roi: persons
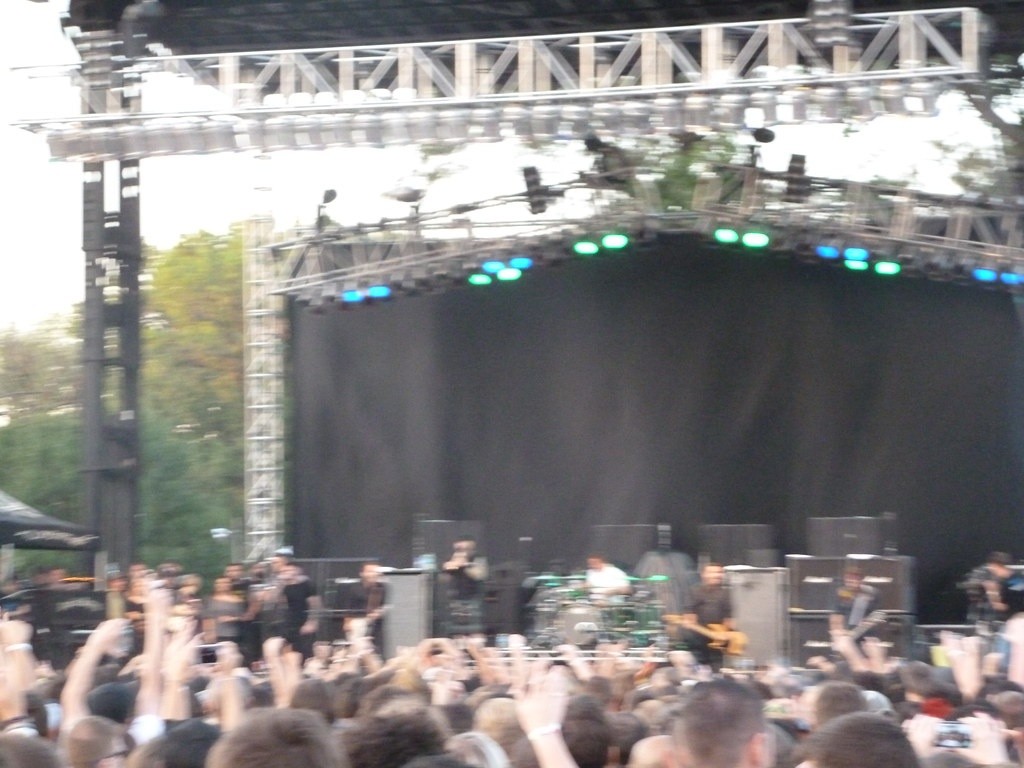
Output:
[829,566,884,645]
[0,537,1024,768]
[439,541,490,645]
[570,553,633,611]
[672,563,740,670]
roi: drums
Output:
[551,603,603,647]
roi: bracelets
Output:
[527,724,563,739]
[1,715,36,724]
[1,723,39,733]
[4,644,34,652]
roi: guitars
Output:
[831,610,889,655]
[661,614,750,655]
